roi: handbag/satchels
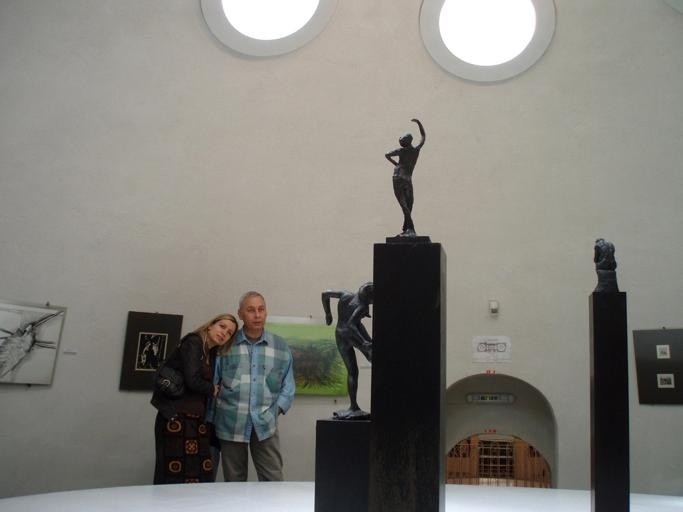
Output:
[153,364,184,399]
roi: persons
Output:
[591,236,617,274]
[205,289,296,484]
[148,313,239,487]
[320,280,371,422]
[381,115,425,240]
